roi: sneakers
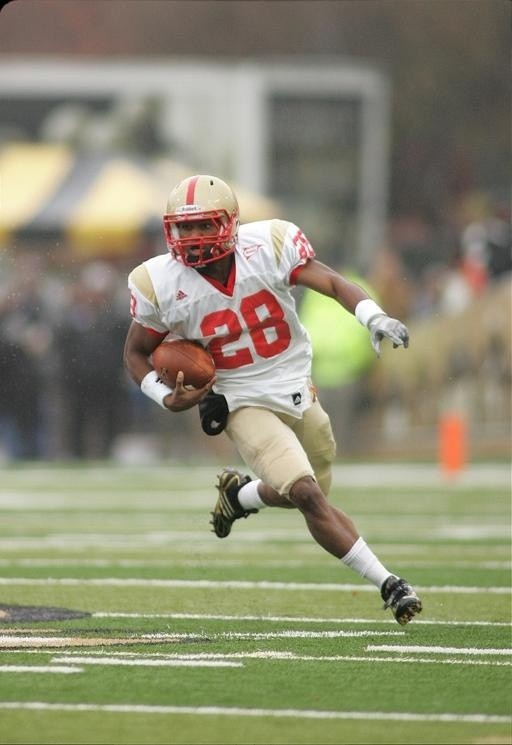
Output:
[380,576,422,627]
[209,467,259,538]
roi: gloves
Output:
[366,312,410,360]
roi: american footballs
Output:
[151,338,215,391]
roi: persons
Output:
[122,175,424,625]
[0,206,512,467]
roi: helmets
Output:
[163,175,240,268]
